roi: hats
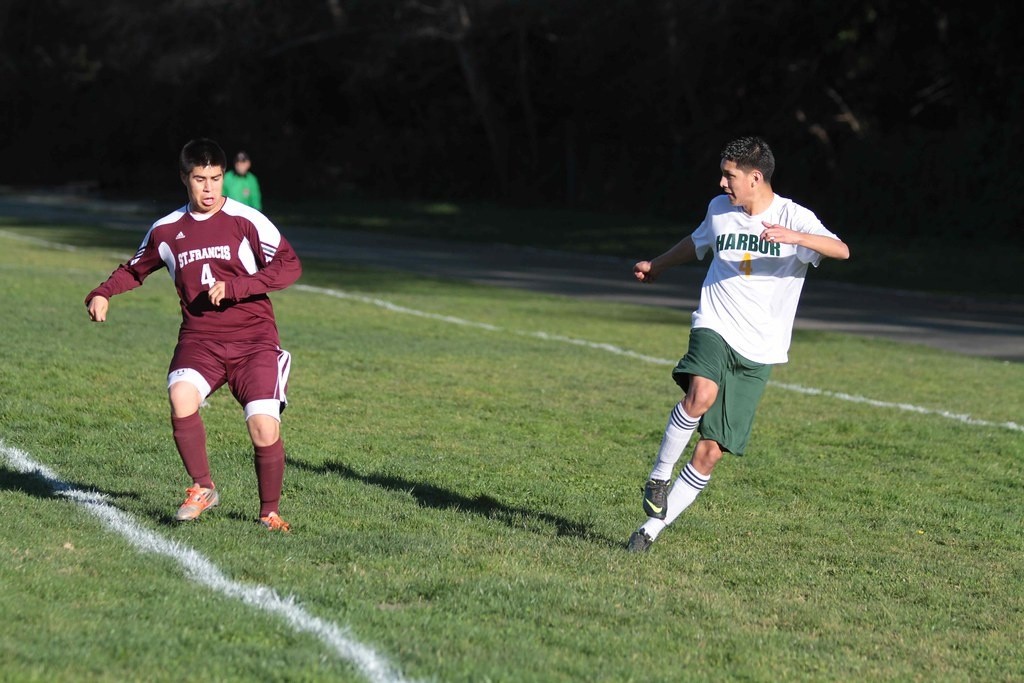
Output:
[236,152,247,160]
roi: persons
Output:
[85,139,301,532]
[221,154,263,210]
[628,137,852,547]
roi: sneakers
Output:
[174,482,219,520]
[643,478,672,519]
[259,512,289,531]
[625,528,653,552]
